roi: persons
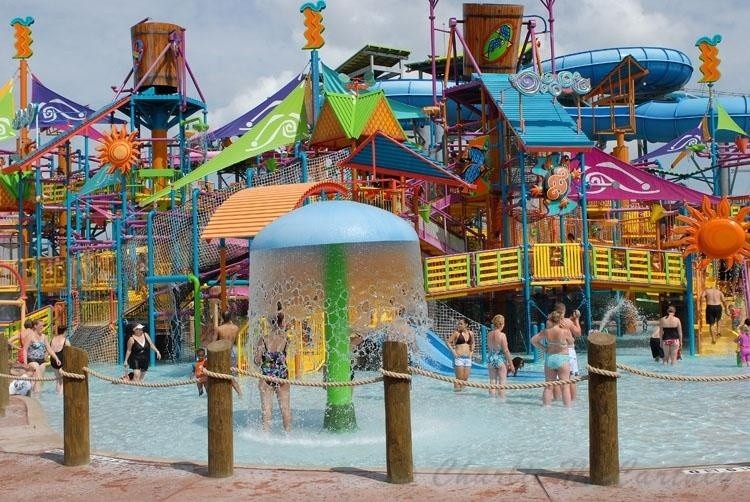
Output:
[189,349,209,396]
[8,366,37,397]
[21,319,62,392]
[649,285,750,368]
[530,310,572,405]
[506,357,524,377]
[0,157,6,170]
[447,317,475,393]
[568,233,575,243]
[8,319,35,364]
[212,309,244,397]
[253,312,291,432]
[119,365,133,382]
[546,303,582,399]
[486,314,515,399]
[50,324,71,394]
[123,323,162,382]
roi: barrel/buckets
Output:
[132,22,184,95]
[463,2,524,77]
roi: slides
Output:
[363,45,744,145]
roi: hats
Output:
[132,324,145,331]
